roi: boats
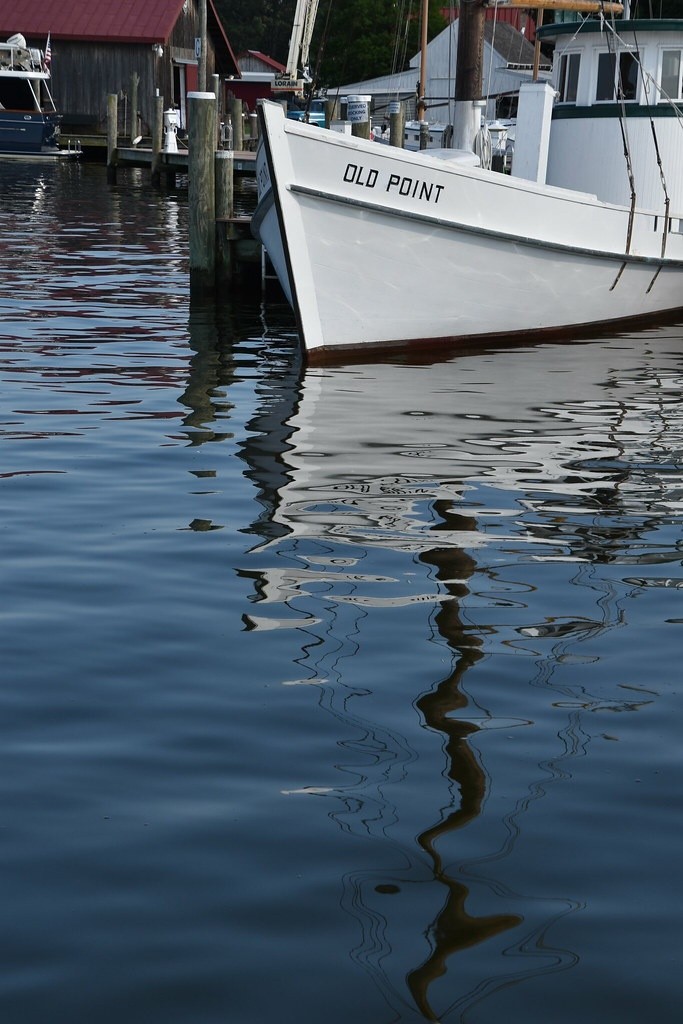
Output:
[236,300,683,634]
[250,0,683,353]
[0,30,88,161]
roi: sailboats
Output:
[370,0,531,171]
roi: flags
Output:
[45,38,51,63]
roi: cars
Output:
[288,98,330,128]
